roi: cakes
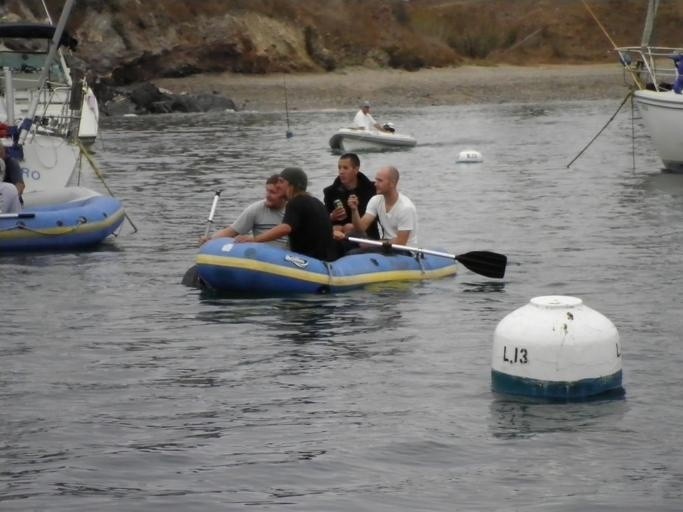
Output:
[333,198,344,209]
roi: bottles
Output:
[359,100,370,108]
[278,167,308,192]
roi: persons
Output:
[321,152,378,249]
[352,101,387,133]
[0,158,21,214]
[232,167,334,261]
[0,142,25,208]
[197,173,287,248]
[346,164,417,255]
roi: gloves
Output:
[0,186,125,250]
[0,0,99,192]
[609,0,683,173]
[196,236,457,298]
[329,127,416,152]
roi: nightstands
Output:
[342,236,507,278]
